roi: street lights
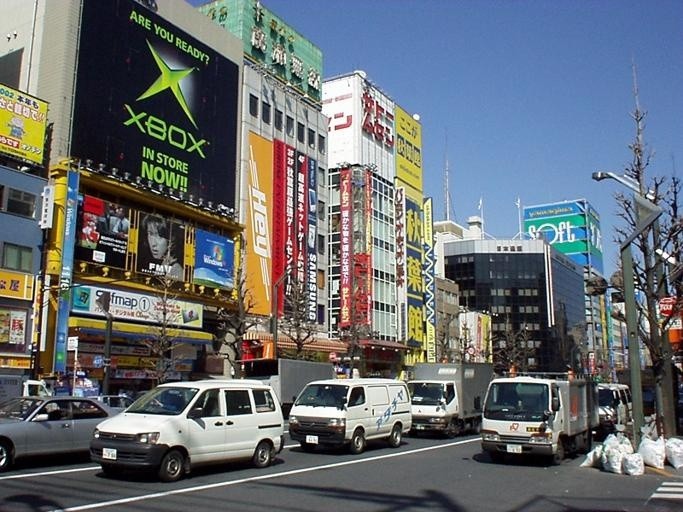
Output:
[591,168,678,448]
[446,309,468,348]
[272,263,304,358]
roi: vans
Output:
[596,382,633,427]
[88,377,287,482]
[288,378,413,456]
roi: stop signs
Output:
[329,352,336,360]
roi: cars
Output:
[82,394,135,414]
[0,395,120,473]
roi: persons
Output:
[111,207,128,234]
[140,213,183,281]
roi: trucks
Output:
[0,374,51,412]
[405,361,497,439]
[477,375,601,468]
[238,357,335,415]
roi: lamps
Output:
[71,263,224,303]
